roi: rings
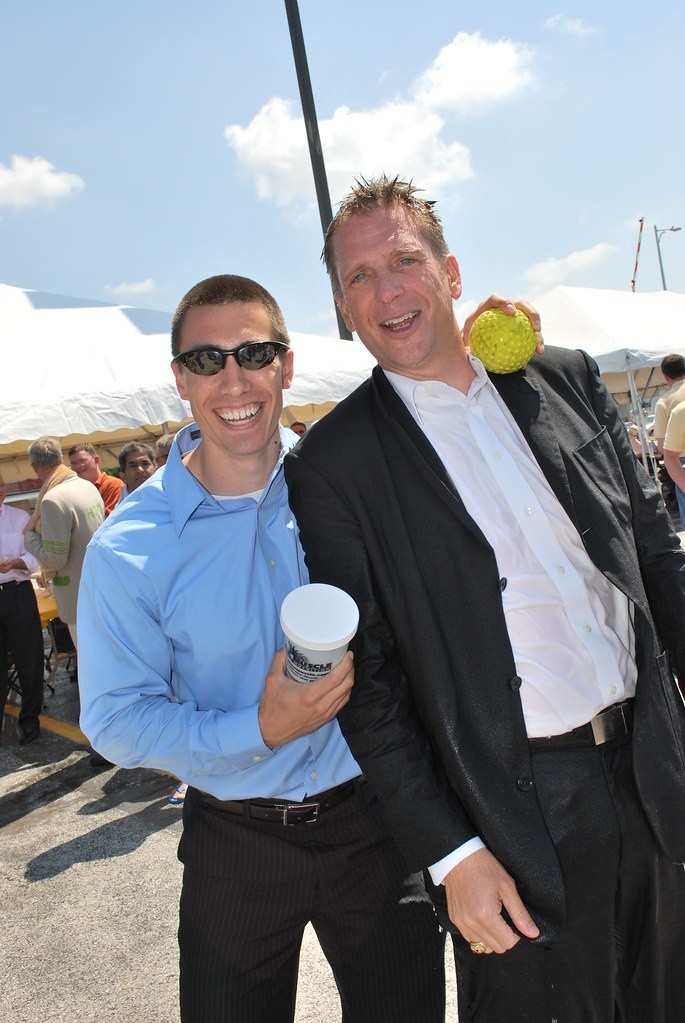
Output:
[470,941,487,954]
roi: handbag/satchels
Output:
[48,617,75,653]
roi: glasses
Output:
[151,455,168,466]
[67,457,94,470]
[173,343,290,377]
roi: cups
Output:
[280,584,362,684]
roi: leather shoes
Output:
[20,728,39,744]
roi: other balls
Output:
[467,306,539,375]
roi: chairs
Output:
[6,615,77,709]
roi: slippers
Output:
[167,785,188,803]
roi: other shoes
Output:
[91,753,109,766]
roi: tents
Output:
[530,286,685,480]
[0,281,380,484]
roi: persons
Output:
[286,175,684,1023]
[0,423,307,805]
[74,273,545,1023]
[629,354,685,529]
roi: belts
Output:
[199,776,367,828]
[0,580,29,591]
[529,699,637,748]
